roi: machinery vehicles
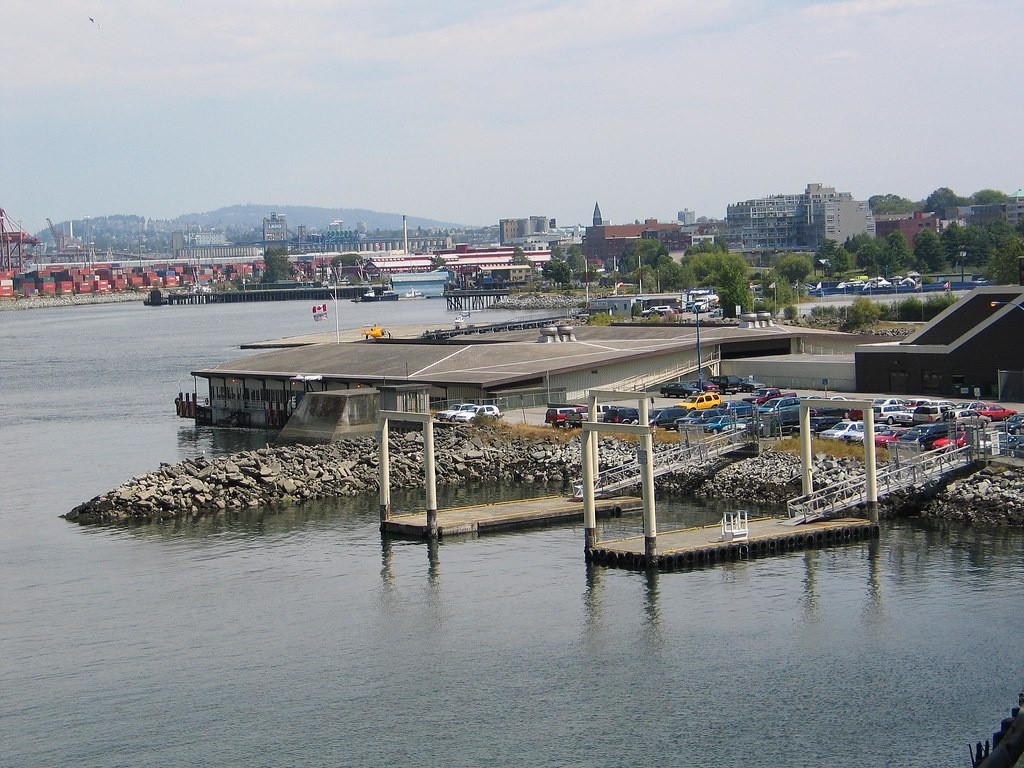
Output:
[693,295,721,312]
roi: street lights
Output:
[579,254,590,309]
[675,297,721,392]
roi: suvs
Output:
[641,305,675,317]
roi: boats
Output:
[351,284,430,303]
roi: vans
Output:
[685,300,695,313]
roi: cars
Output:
[434,403,503,424]
[544,374,1024,458]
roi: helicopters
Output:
[454,311,471,325]
[361,323,386,339]
